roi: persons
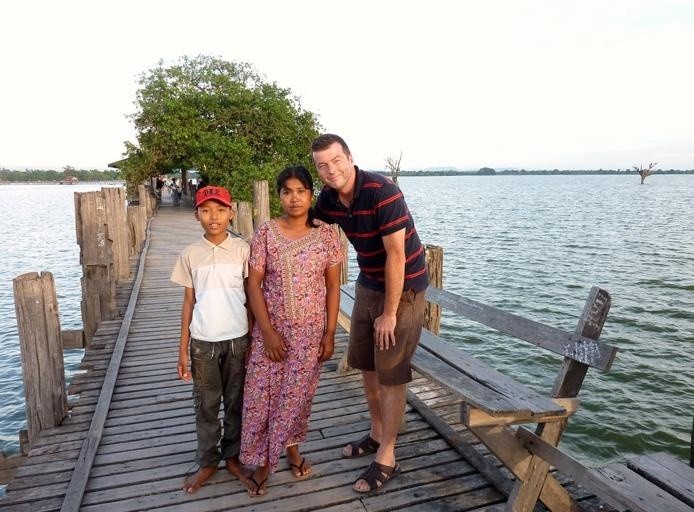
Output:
[187,180,191,187]
[155,177,165,194]
[169,176,181,206]
[305,133,433,495]
[242,165,344,499]
[168,185,255,499]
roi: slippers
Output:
[352,458,400,493]
[289,458,310,477]
[342,432,381,458]
[247,473,267,496]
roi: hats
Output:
[195,186,231,207]
